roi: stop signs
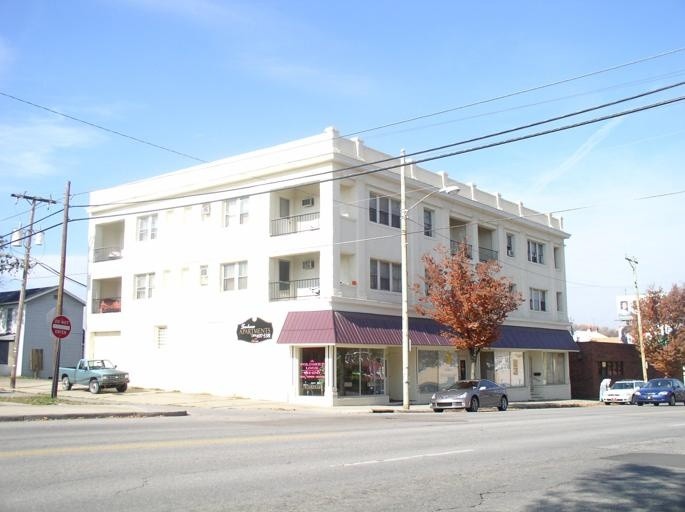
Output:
[51,315,72,338]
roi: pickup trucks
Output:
[58,358,131,395]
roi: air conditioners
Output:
[301,198,313,207]
[302,259,313,270]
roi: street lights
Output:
[399,185,462,409]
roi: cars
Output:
[419,381,445,392]
[429,378,509,413]
[602,377,685,407]
